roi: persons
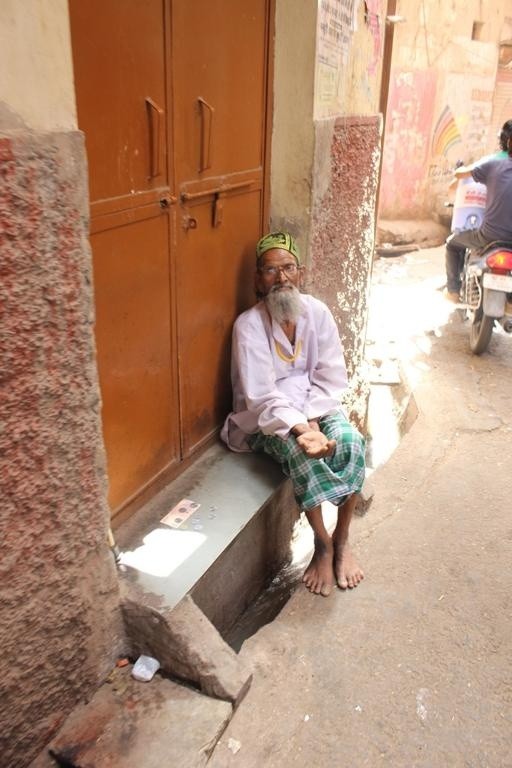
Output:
[443,124,512,319]
[218,230,368,599]
[447,118,512,190]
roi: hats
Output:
[255,231,301,268]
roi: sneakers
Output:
[504,302,512,317]
[446,293,460,303]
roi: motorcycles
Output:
[437,159,512,354]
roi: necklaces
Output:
[275,341,302,362]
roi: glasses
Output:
[260,265,298,274]
